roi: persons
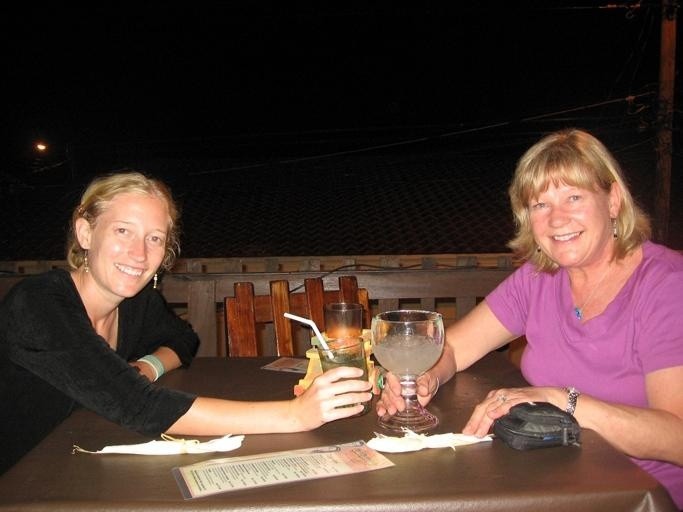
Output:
[0,173,374,471]
[375,130,683,512]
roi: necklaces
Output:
[572,258,612,320]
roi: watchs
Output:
[564,385,581,416]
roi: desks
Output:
[0,359,668,512]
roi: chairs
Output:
[224,276,368,360]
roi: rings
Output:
[496,395,506,401]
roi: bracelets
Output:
[136,354,164,383]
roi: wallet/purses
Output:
[489,400,581,450]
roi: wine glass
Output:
[369,309,445,437]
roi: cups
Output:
[323,302,362,340]
[317,336,372,419]
